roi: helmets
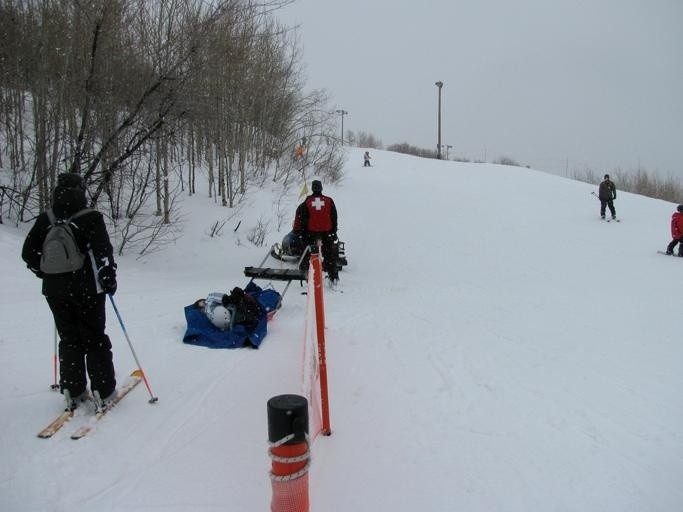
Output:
[677,204,683,211]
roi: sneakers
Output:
[67,383,86,401]
[89,380,117,400]
[328,271,339,282]
[601,215,616,219]
[666,249,683,257]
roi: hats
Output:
[311,180,323,191]
[56,172,86,191]
[604,174,609,179]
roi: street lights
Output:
[446,144,451,159]
[434,80,444,159]
[334,109,350,146]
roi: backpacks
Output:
[39,207,102,275]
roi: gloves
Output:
[27,258,43,279]
[97,258,117,296]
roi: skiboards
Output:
[36,369,144,440]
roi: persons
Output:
[21,172,117,410]
[293,180,340,285]
[666,205,683,257]
[364,152,372,166]
[194,287,264,333]
[599,175,616,219]
